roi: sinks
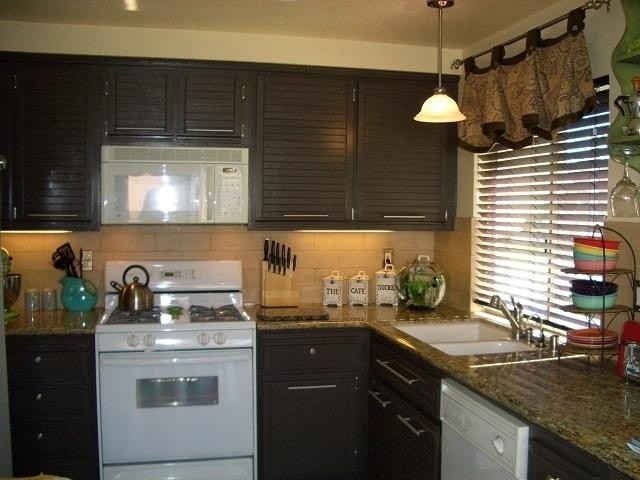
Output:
[431,342,539,356]
[393,321,512,342]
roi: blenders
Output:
[0,246,24,319]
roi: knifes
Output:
[262,237,297,274]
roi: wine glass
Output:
[608,146,640,217]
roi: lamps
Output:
[411,1,467,123]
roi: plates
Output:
[566,328,618,349]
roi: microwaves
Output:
[101,144,249,225]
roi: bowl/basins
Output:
[567,277,618,309]
[571,236,621,270]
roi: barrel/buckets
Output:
[615,320,640,382]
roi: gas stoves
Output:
[95,294,256,352]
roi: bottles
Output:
[25,287,57,313]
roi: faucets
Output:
[525,313,545,347]
[489,296,528,339]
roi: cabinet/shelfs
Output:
[526,422,639,480]
[256,329,364,480]
[1,50,102,234]
[547,216,638,376]
[101,54,252,148]
[605,0,640,174]
[363,334,440,479]
[4,331,95,480]
[251,61,460,232]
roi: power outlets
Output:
[385,254,392,265]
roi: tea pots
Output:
[109,263,155,310]
[614,74,640,135]
[58,275,98,311]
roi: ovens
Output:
[98,345,257,480]
